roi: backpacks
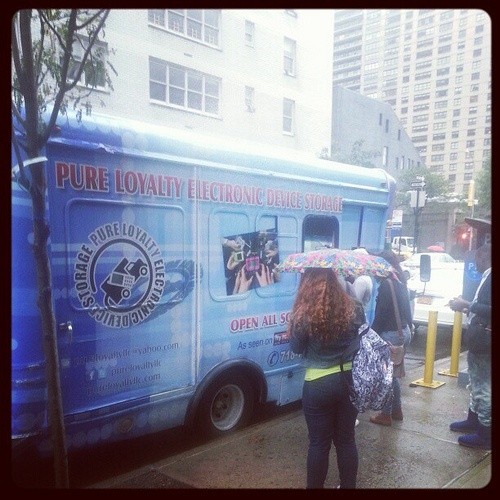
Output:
[339,299,395,412]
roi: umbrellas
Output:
[428,246,445,251]
[465,217,491,233]
[274,249,399,280]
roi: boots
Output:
[449,407,481,433]
[458,421,492,448]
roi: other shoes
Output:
[391,407,404,420]
[370,412,391,425]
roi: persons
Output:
[446,244,491,448]
[339,250,413,426]
[287,266,366,489]
[223,236,278,295]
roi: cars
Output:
[387,235,421,257]
[408,261,474,343]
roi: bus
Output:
[9,103,398,465]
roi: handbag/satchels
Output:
[389,344,406,378]
[463,323,492,357]
